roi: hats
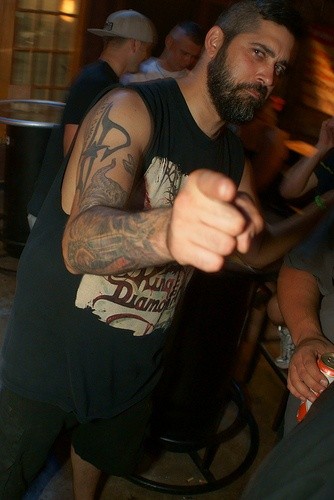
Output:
[87,9,155,42]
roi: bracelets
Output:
[315,195,325,209]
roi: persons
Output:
[0,0,309,500]
[27,9,157,231]
[239,208,334,500]
[120,22,205,85]
[227,93,291,186]
[272,116,334,221]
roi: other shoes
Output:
[275,324,295,369]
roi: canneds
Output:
[295,353,334,425]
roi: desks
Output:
[0,100,66,276]
[128,199,287,494]
